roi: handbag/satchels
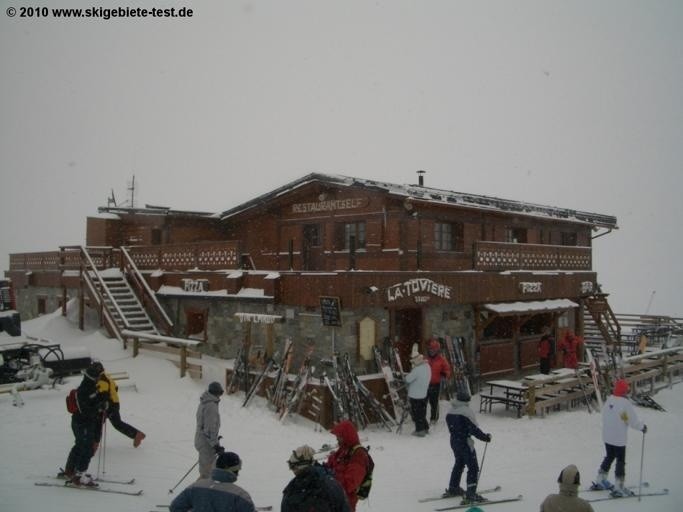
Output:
[65,389,83,417]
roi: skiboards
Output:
[155,504,273,511]
[575,329,673,415]
[226,336,473,433]
[34,478,143,495]
[419,486,523,512]
[579,482,669,503]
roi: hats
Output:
[409,352,423,365]
[288,444,316,476]
[427,340,441,353]
[208,381,224,396]
[215,451,243,473]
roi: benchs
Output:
[480,350,683,420]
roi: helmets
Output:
[612,377,627,397]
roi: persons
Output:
[445,392,491,501]
[194,382,224,477]
[638,329,649,352]
[422,339,452,423]
[557,329,584,368]
[324,420,374,512]
[540,465,596,512]
[281,445,353,512]
[169,452,257,512]
[90,361,145,455]
[537,326,557,374]
[65,365,111,485]
[595,380,649,498]
[401,351,432,437]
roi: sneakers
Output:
[63,470,97,486]
[612,486,635,498]
[409,429,430,438]
[463,492,488,502]
[445,486,465,495]
[594,477,613,490]
[133,429,145,447]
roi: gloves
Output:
[642,425,647,434]
[485,433,492,442]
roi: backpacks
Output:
[344,444,374,501]
